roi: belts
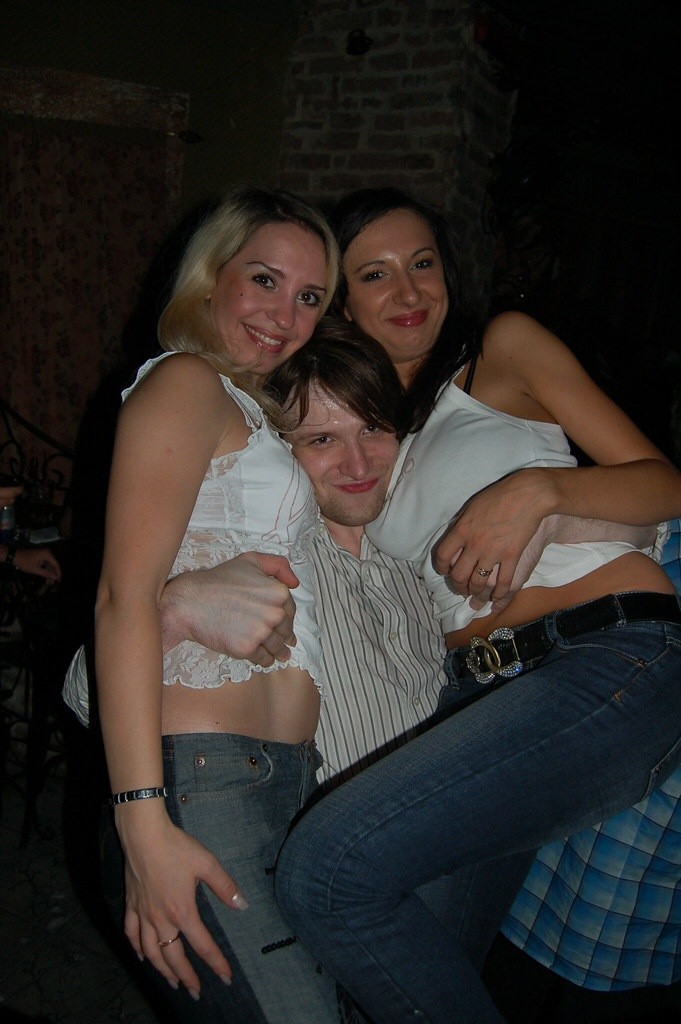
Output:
[452,593,681,684]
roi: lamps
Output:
[346,29,373,55]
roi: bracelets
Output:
[110,787,168,806]
[5,543,17,566]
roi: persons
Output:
[60,182,681,1024]
[0,486,62,581]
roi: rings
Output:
[474,566,492,577]
[158,934,180,946]
